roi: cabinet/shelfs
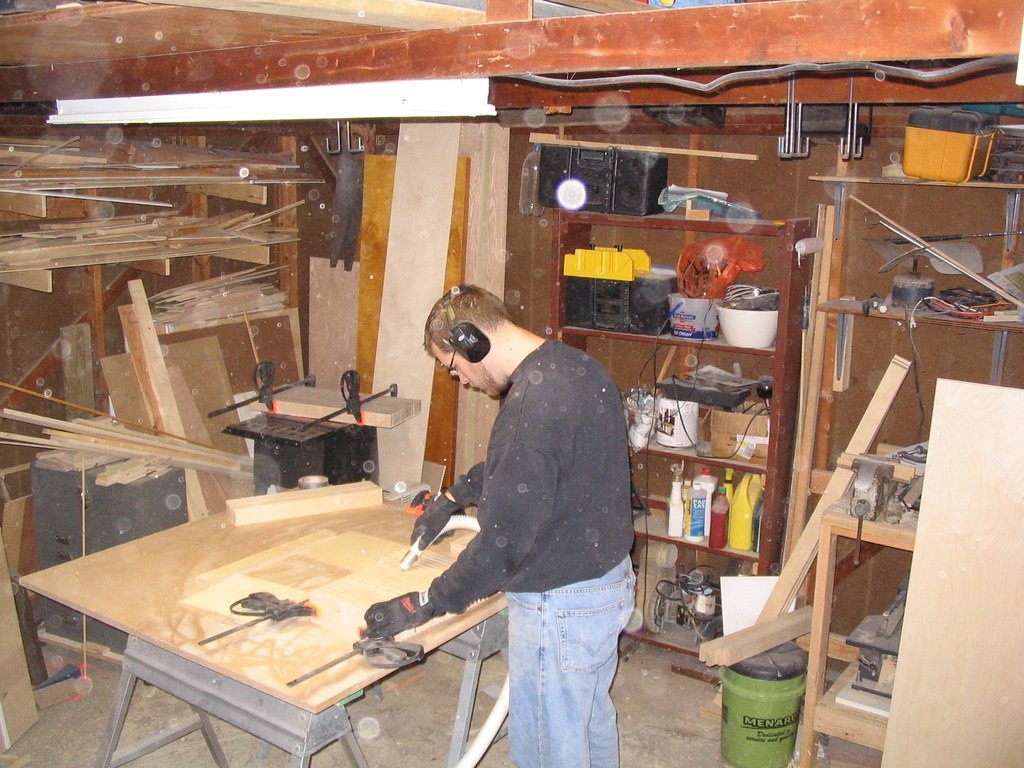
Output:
[546,209,812,657]
[794,105,1024,663]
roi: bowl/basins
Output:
[716,303,778,350]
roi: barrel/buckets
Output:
[669,292,722,341]
[719,641,809,768]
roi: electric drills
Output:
[286,628,424,692]
[196,590,322,647]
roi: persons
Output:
[362,283,637,768]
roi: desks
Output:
[18,484,509,768]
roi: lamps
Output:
[47,78,497,123]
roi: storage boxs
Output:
[902,105,1000,183]
[712,401,770,465]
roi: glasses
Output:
[448,350,460,377]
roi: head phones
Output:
[440,290,490,363]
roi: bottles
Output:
[669,462,767,552]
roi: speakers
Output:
[539,146,575,208]
[629,274,678,336]
[565,275,595,329]
[612,150,668,217]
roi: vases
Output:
[716,303,779,348]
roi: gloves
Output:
[409,493,460,551]
[363,587,435,638]
